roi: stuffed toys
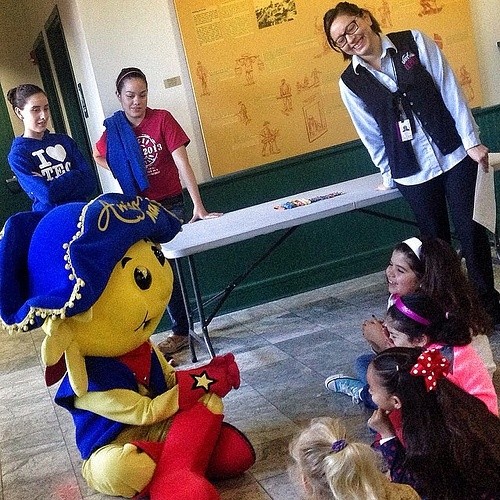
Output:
[1,195,256,500]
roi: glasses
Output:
[333,15,359,49]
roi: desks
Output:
[160,153,500,370]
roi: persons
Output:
[6,83,98,214]
[325,235,489,407]
[366,347,500,500]
[383,294,499,416]
[92,68,224,353]
[289,417,421,500]
[324,3,500,333]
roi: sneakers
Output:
[325,375,367,403]
[158,333,194,354]
